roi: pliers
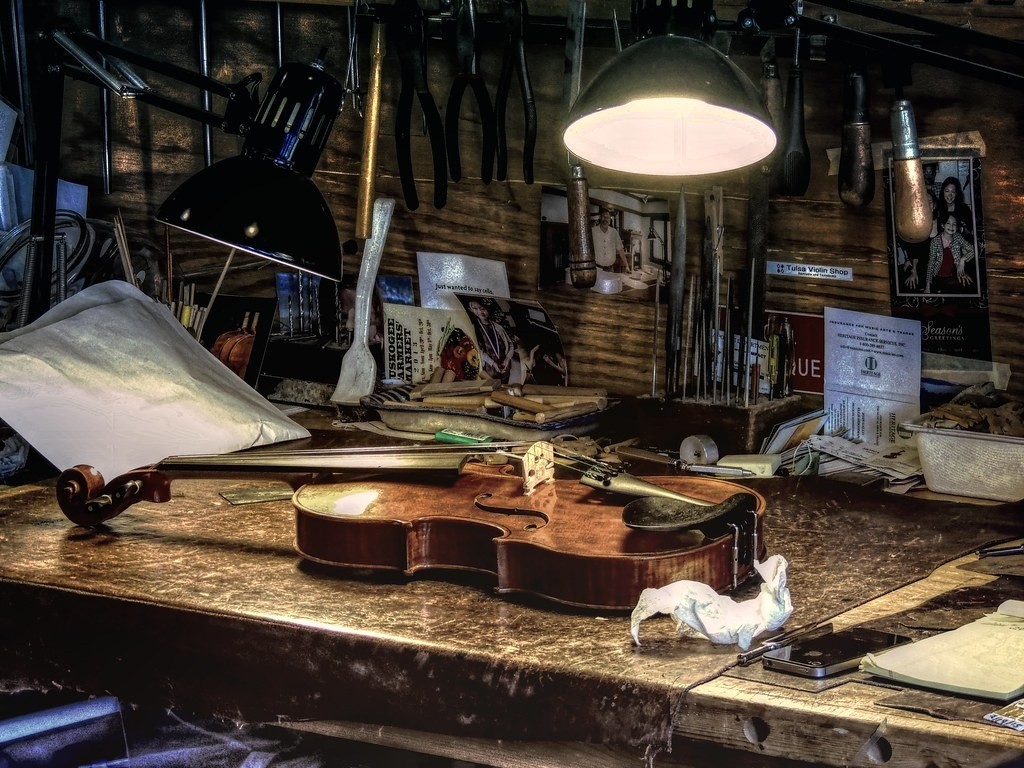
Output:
[446,0,495,185]
[391,0,448,214]
[494,0,539,186]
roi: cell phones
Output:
[760,626,915,678]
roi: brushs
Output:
[330,194,397,421]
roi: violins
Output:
[57,442,768,611]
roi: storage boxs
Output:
[902,410,1024,503]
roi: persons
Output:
[592,207,632,274]
[466,296,566,386]
[896,162,976,295]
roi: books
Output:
[858,599,1024,700]
[716,455,782,476]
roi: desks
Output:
[2,413,1023,768]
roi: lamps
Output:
[0,0,345,485]
[562,1,1024,177]
